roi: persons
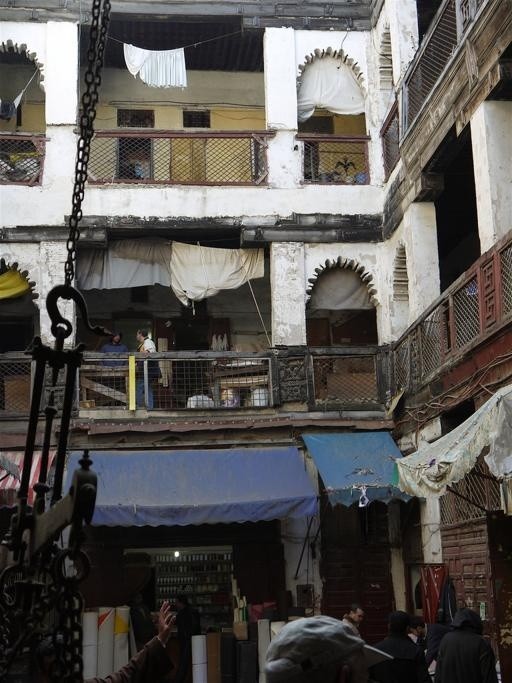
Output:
[99,330,128,405]
[175,594,201,683]
[342,603,499,683]
[136,329,157,410]
[28,601,176,683]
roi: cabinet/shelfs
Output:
[127,548,235,627]
